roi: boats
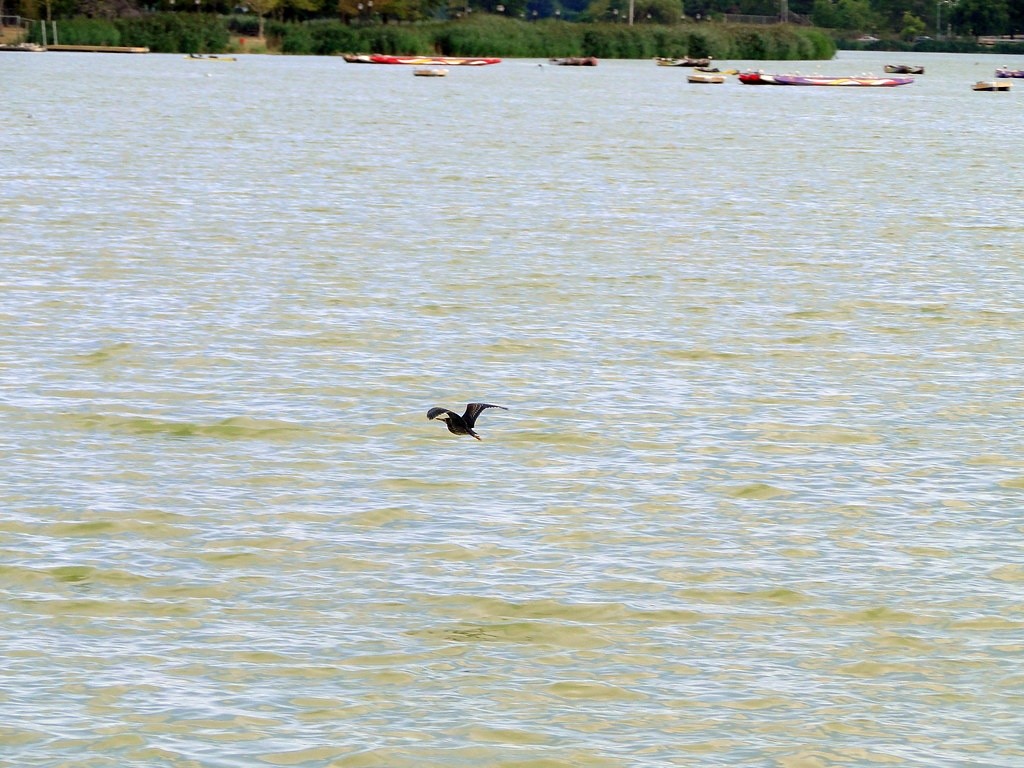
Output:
[994,67,1024,80]
[969,76,1013,91]
[884,64,925,75]
[738,71,915,88]
[654,54,721,73]
[685,73,727,85]
[556,56,597,68]
[343,52,502,68]
[412,67,450,76]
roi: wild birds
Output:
[426,403,510,448]
